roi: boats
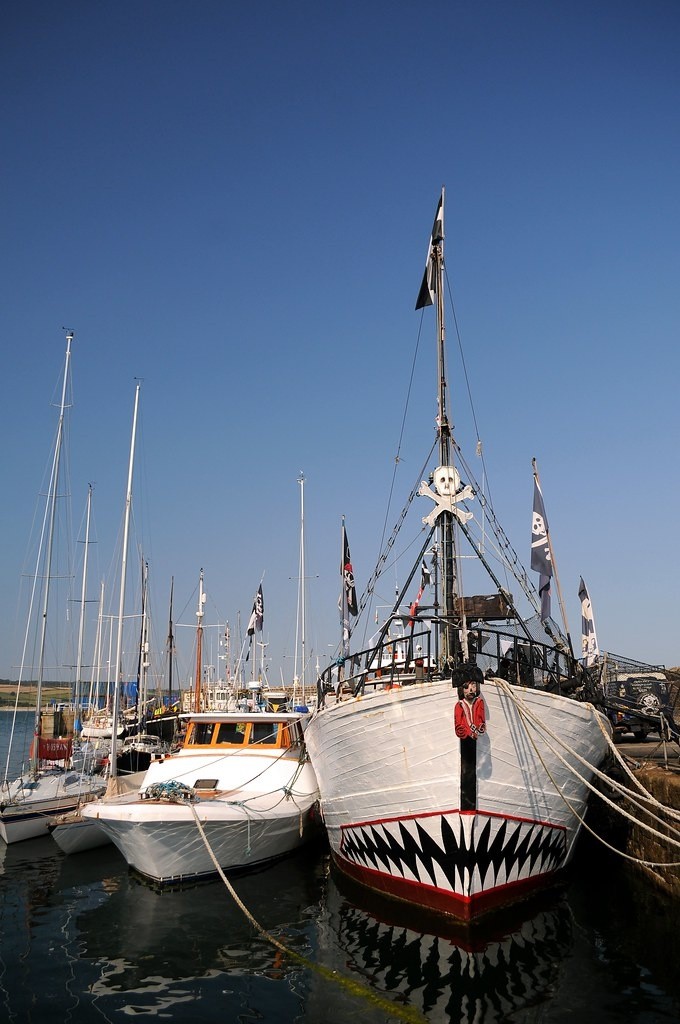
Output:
[72,708,323,885]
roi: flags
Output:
[531,480,553,620]
[422,561,431,587]
[340,531,357,615]
[247,583,263,634]
[415,195,443,310]
[579,578,599,662]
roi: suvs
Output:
[605,671,671,744]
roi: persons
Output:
[452,664,485,738]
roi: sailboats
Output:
[285,178,620,933]
[299,850,613,1024]
[0,317,256,854]
